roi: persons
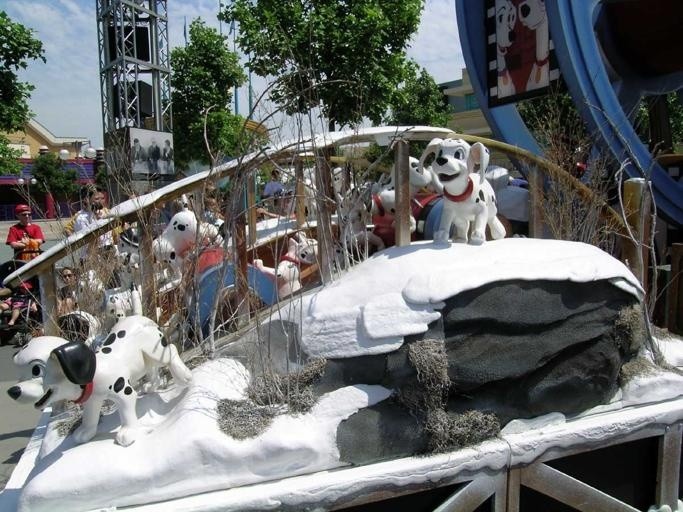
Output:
[73,185,121,289]
[130,138,145,165]
[5,204,45,262]
[54,268,83,309]
[148,168,311,233]
[145,139,160,172]
[159,139,172,172]
[0,281,38,325]
[61,184,124,289]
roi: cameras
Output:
[87,201,104,212]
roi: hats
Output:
[14,204,30,215]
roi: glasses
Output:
[19,213,31,216]
